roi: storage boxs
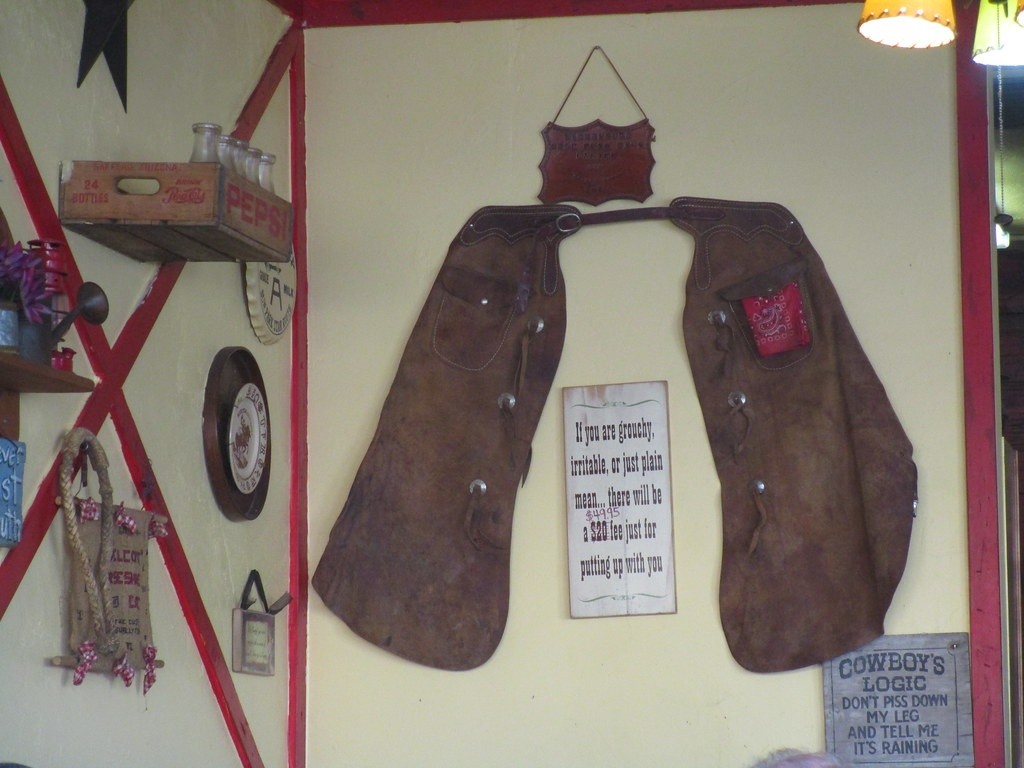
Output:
[52,156,292,265]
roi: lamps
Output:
[857,0,959,48]
[973,0,1023,68]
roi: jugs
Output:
[0,282,110,373]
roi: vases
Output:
[0,301,22,346]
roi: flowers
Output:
[0,241,61,323]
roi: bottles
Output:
[236,139,249,179]
[189,120,222,164]
[247,148,263,188]
[220,135,236,171]
[259,153,276,195]
